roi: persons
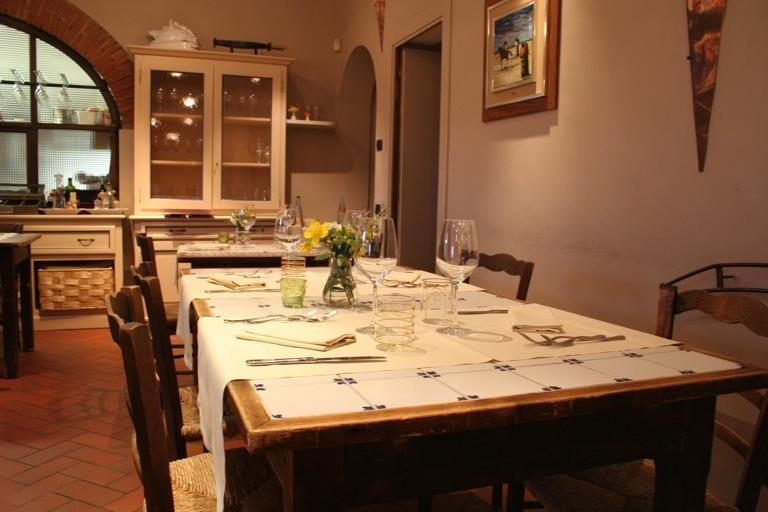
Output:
[498,40,509,69]
[512,38,520,58]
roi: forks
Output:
[515,327,586,347]
[198,265,339,325]
[242,326,359,351]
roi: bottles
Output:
[294,196,301,220]
[97,183,109,209]
[338,196,346,223]
[65,178,77,209]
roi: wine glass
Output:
[249,137,271,163]
[353,218,398,334]
[153,83,269,116]
[229,204,256,250]
[435,218,479,336]
[273,209,303,256]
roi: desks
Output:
[0,232,42,380]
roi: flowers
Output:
[298,218,371,305]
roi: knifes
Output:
[458,308,509,319]
[246,354,386,367]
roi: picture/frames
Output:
[481,0,559,124]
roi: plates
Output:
[37,207,85,215]
[85,207,128,215]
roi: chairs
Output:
[105,287,380,512]
[133,235,197,390]
[376,251,532,449]
[506,264,767,509]
[124,260,226,461]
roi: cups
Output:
[216,231,227,247]
[94,198,110,210]
[372,293,416,348]
[421,276,457,330]
[280,256,306,309]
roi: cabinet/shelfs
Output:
[129,213,294,327]
[19,220,125,332]
[125,42,297,215]
